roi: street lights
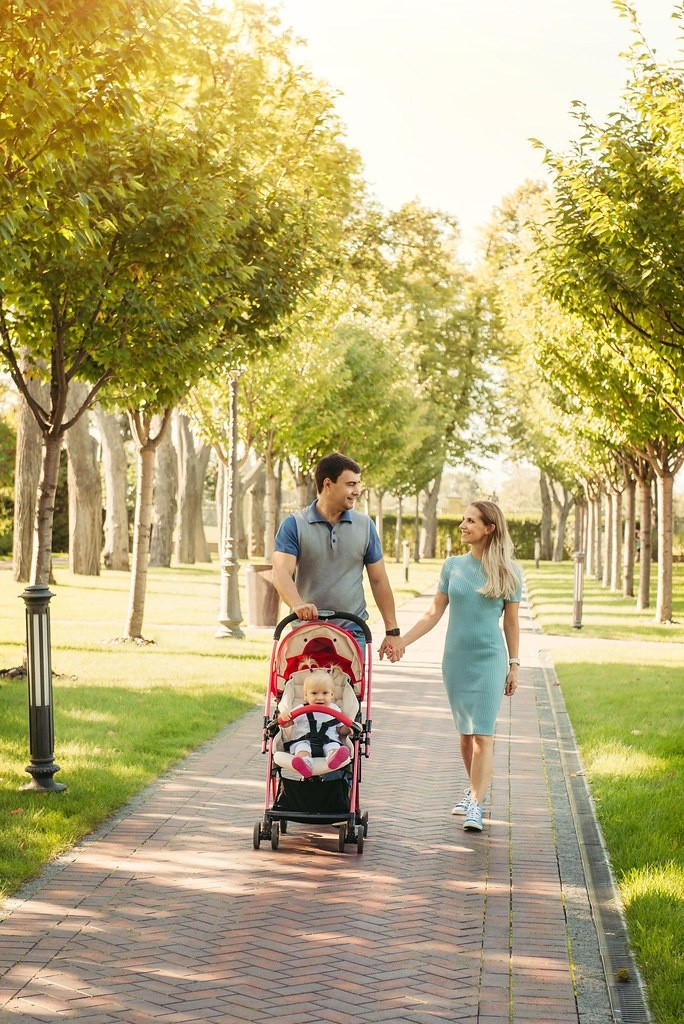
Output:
[215,365,247,637]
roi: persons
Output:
[271,451,405,830]
[278,668,364,779]
[377,501,523,832]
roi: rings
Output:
[303,614,308,618]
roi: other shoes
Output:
[292,756,312,778]
[327,746,349,769]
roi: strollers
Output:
[253,609,374,857]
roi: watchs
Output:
[385,628,400,636]
[508,657,520,667]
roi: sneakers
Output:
[451,792,486,814]
[463,804,482,831]
